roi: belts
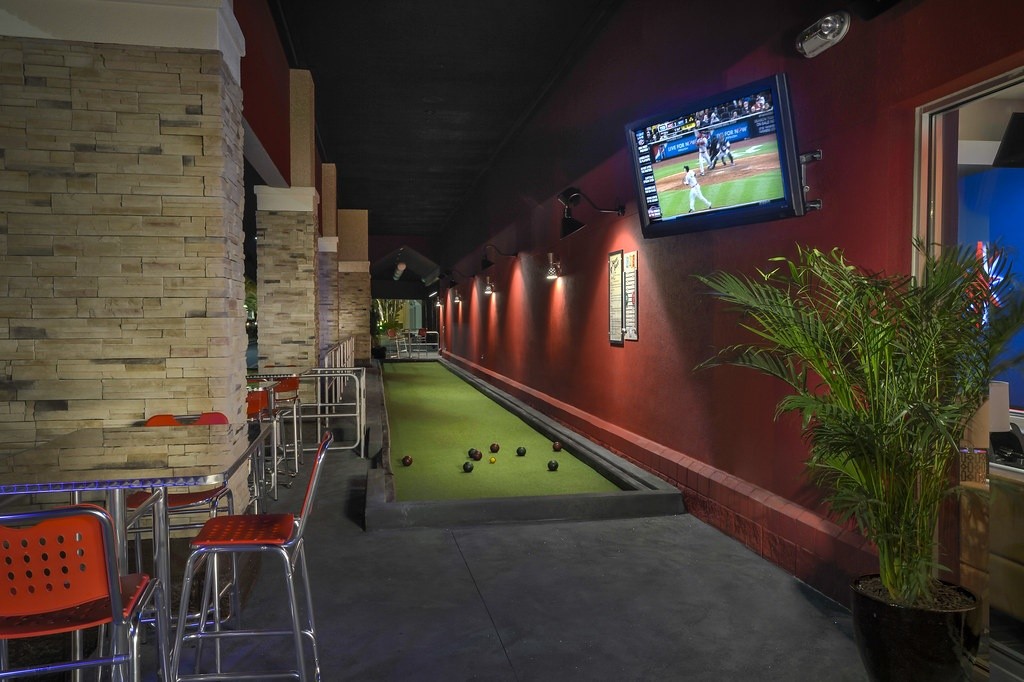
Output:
[692,183,697,188]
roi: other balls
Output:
[547,459,559,471]
[472,450,482,460]
[553,441,562,451]
[516,447,526,456]
[463,462,474,472]
[490,457,496,463]
[402,456,413,466]
[468,448,477,457]
[490,443,500,453]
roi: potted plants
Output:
[686,235,1024,682]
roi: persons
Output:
[372,337,384,373]
[647,131,666,163]
[682,166,711,213]
[695,94,772,133]
[696,131,735,176]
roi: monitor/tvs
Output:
[625,72,806,239]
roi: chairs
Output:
[0,375,334,682]
[385,328,428,358]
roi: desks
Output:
[0,423,275,682]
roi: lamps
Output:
[480,244,517,272]
[393,248,407,282]
[558,191,625,242]
[483,276,495,294]
[435,296,445,307]
[449,269,475,289]
[454,290,463,303]
[546,252,561,280]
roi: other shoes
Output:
[689,210,695,213]
[708,202,712,209]
